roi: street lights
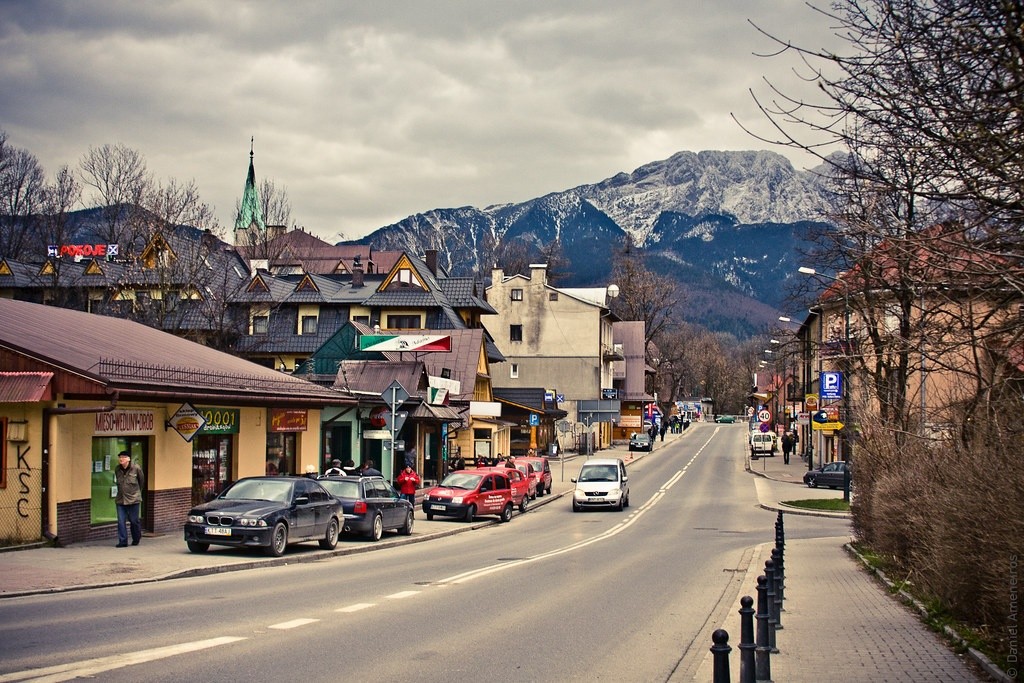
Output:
[769,339,798,455]
[758,365,774,428]
[797,265,853,503]
[779,316,814,471]
[765,350,787,436]
[762,361,779,436]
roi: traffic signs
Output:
[821,371,843,402]
[810,421,847,435]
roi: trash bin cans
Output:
[549,444,557,457]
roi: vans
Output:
[751,433,775,457]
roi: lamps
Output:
[441,368,451,379]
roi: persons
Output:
[325,460,346,476]
[115,451,145,548]
[476,454,487,467]
[663,420,680,434]
[505,456,516,468]
[278,451,288,472]
[355,460,380,474]
[397,464,420,506]
[630,430,637,438]
[648,422,665,442]
[492,453,504,466]
[524,448,535,457]
[781,431,796,465]
[343,460,357,474]
[451,452,465,470]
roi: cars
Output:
[628,433,654,451]
[644,421,653,432]
[313,473,417,541]
[749,430,779,449]
[422,469,517,522]
[716,415,736,425]
[496,461,540,504]
[182,475,345,559]
[570,458,631,512]
[801,460,857,491]
[515,456,554,497]
[479,467,531,512]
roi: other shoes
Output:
[116,543,128,547]
[132,535,141,546]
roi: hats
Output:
[404,462,412,468]
[118,451,131,457]
[331,459,342,463]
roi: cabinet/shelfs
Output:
[193,434,231,503]
[266,432,297,474]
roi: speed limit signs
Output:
[758,410,776,424]
